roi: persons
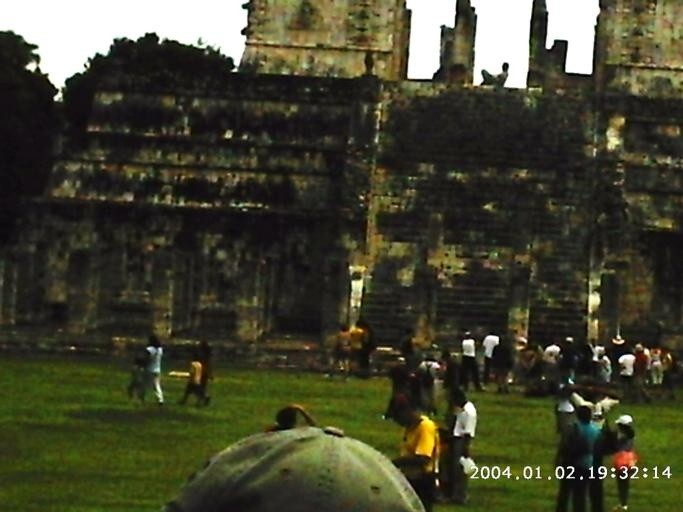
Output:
[383,328,479,512]
[480,63,508,85]
[331,322,352,382]
[553,373,639,512]
[196,340,209,389]
[127,361,144,401]
[178,348,209,406]
[133,334,164,405]
[462,331,676,405]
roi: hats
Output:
[385,392,413,418]
[163,424,427,511]
[615,415,634,425]
